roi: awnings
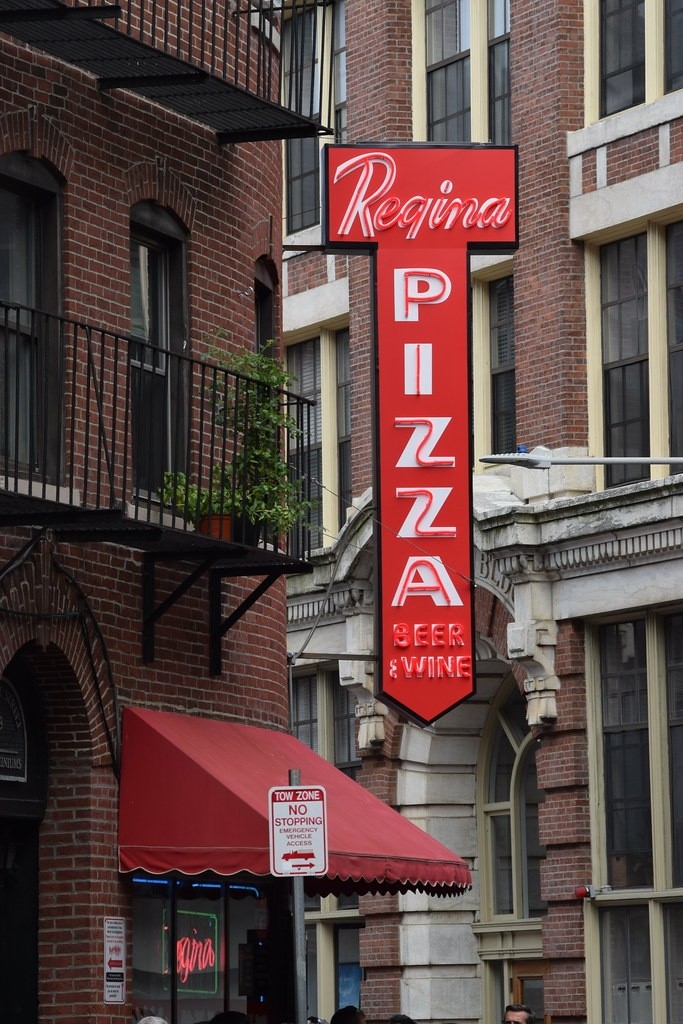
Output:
[119,703,472,898]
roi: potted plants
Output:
[160,327,315,548]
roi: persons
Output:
[133,1002,539,1023]
